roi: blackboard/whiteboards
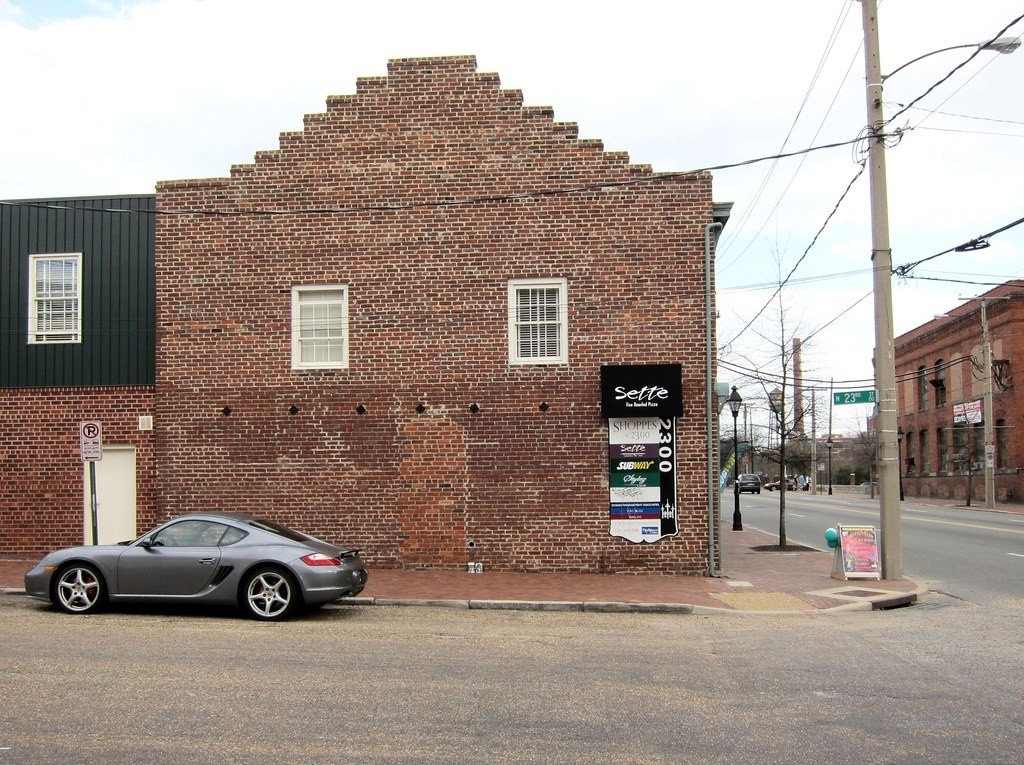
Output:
[831,524,880,577]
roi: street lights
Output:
[825,436,832,495]
[725,385,743,531]
[865,35,1024,580]
[896,426,904,501]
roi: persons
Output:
[799,474,812,491]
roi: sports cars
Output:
[25,510,369,622]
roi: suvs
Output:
[736,473,761,494]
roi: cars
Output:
[763,478,809,492]
